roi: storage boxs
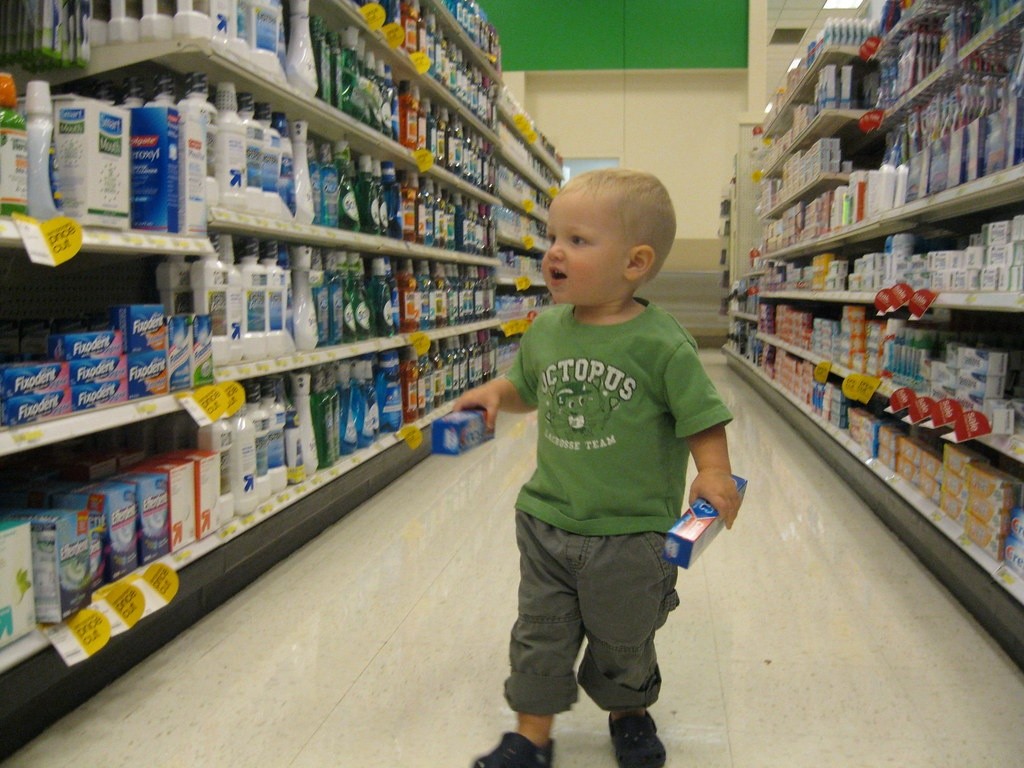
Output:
[430,408,496,455]
[661,474,749,570]
[768,220,1024,580]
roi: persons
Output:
[452,170,741,768]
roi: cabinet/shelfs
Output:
[0,0,563,768]
[719,0,1024,670]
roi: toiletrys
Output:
[0,1,497,651]
[497,79,565,380]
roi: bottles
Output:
[25,80,64,220]
[98,0,498,522]
[0,73,28,218]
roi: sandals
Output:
[609,710,666,768]
[474,733,553,768]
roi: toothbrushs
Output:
[867,5,1024,208]
